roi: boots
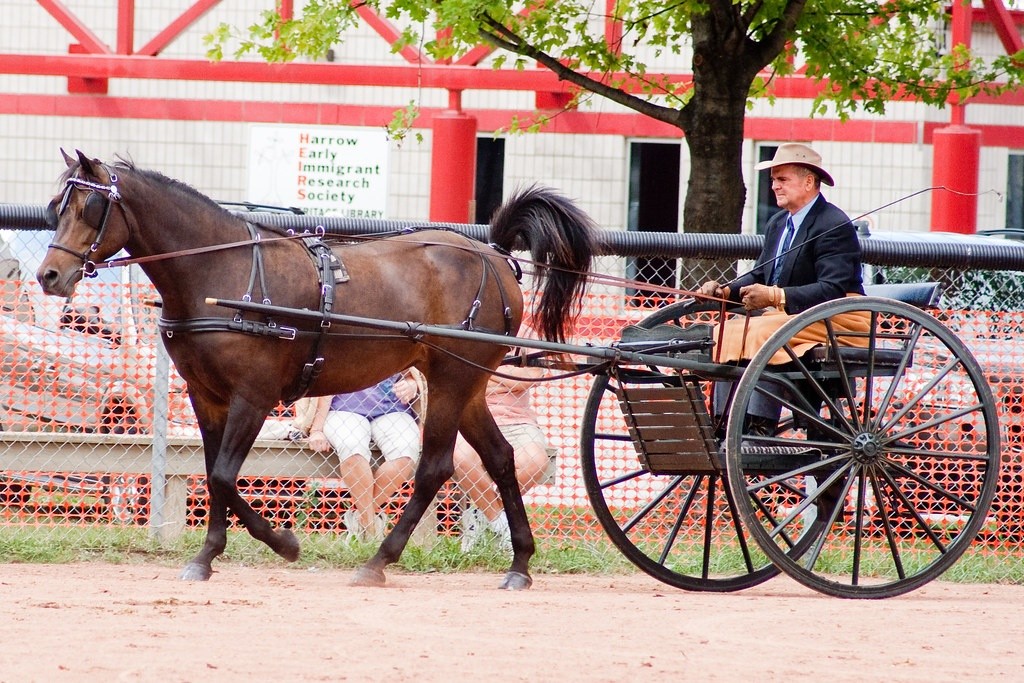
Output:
[713,416,728,452]
[741,416,778,447]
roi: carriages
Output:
[35,148,1001,599]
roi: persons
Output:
[290,320,550,555]
[694,142,864,448]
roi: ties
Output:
[771,216,795,286]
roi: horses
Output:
[33,146,602,588]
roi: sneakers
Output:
[374,512,387,540]
[462,504,484,555]
[344,509,362,546]
[490,511,514,561]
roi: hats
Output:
[753,143,834,186]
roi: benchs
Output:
[0,430,445,549]
[801,281,942,367]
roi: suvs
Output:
[0,199,356,529]
[826,228,1024,547]
